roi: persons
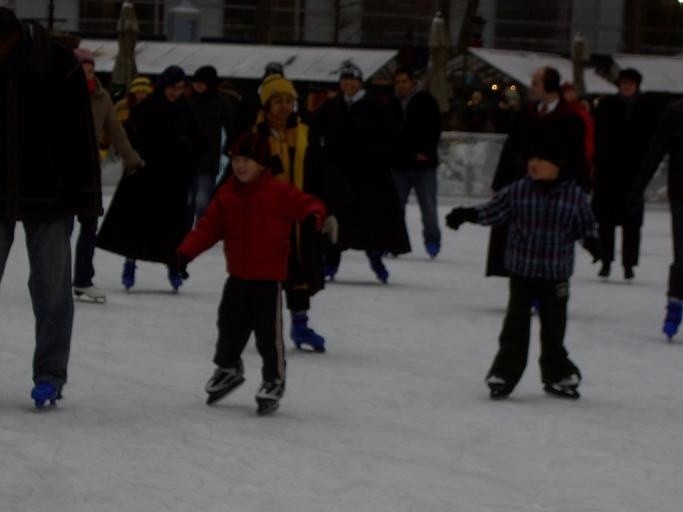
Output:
[311,63,389,285]
[385,72,440,260]
[560,80,594,161]
[443,138,602,401]
[595,66,666,281]
[191,62,219,98]
[458,87,520,131]
[220,72,339,352]
[1,7,101,409]
[485,66,588,279]
[662,93,683,342]
[114,76,154,121]
[96,64,204,294]
[73,51,146,303]
[177,134,337,416]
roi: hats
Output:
[338,61,362,80]
[527,142,563,165]
[157,66,185,87]
[257,75,294,106]
[192,65,218,85]
[620,67,640,81]
[72,48,94,66]
[129,77,154,94]
[263,61,284,76]
[230,134,271,166]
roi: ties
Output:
[346,97,353,110]
[538,104,548,118]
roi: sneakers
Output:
[661,299,682,337]
[487,363,522,392]
[542,360,580,387]
[324,263,337,276]
[290,317,325,348]
[205,361,245,393]
[370,260,388,279]
[426,240,441,255]
[31,382,59,401]
[73,281,105,298]
[168,267,183,289]
[121,263,134,286]
[255,378,285,401]
[624,266,633,278]
[598,263,611,277]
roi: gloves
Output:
[322,214,339,243]
[176,255,188,278]
[445,206,476,230]
[582,237,604,263]
[302,216,316,235]
[629,187,646,209]
